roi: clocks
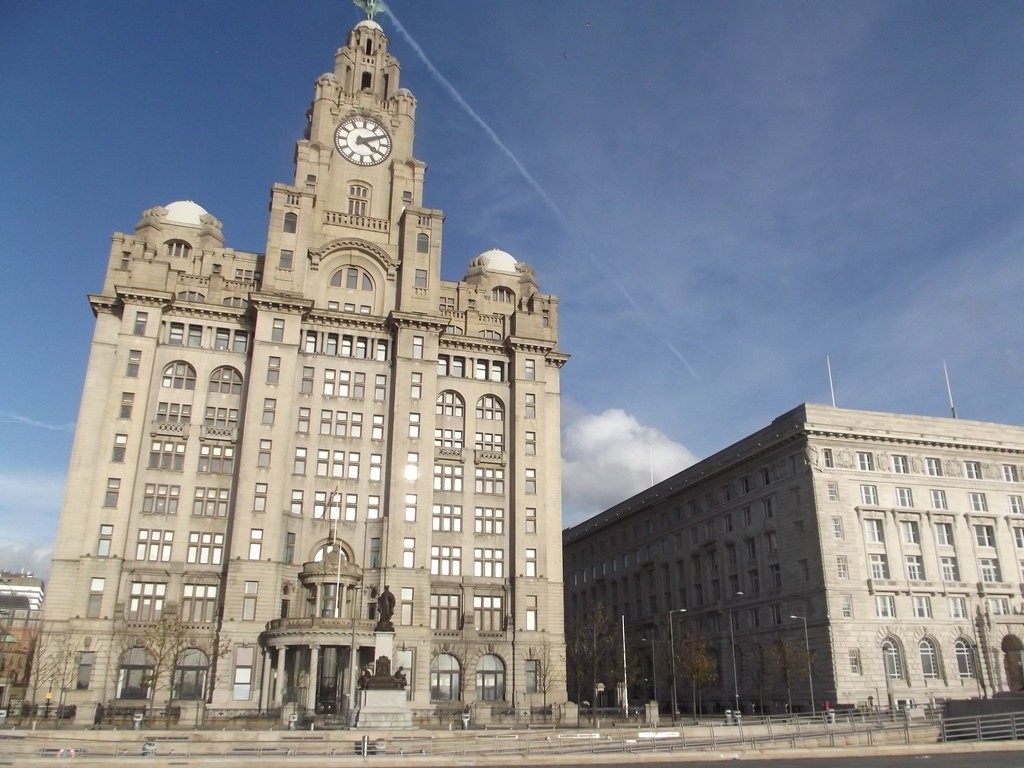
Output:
[334,118,392,167]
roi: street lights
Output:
[670,609,688,721]
[791,615,813,715]
[641,638,657,702]
[730,591,745,712]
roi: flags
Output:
[320,485,339,517]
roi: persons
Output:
[395,666,407,690]
[358,666,371,690]
[374,585,395,632]
[91,702,104,730]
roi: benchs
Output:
[202,704,281,725]
[22,705,78,726]
[432,699,646,724]
[104,704,181,724]
